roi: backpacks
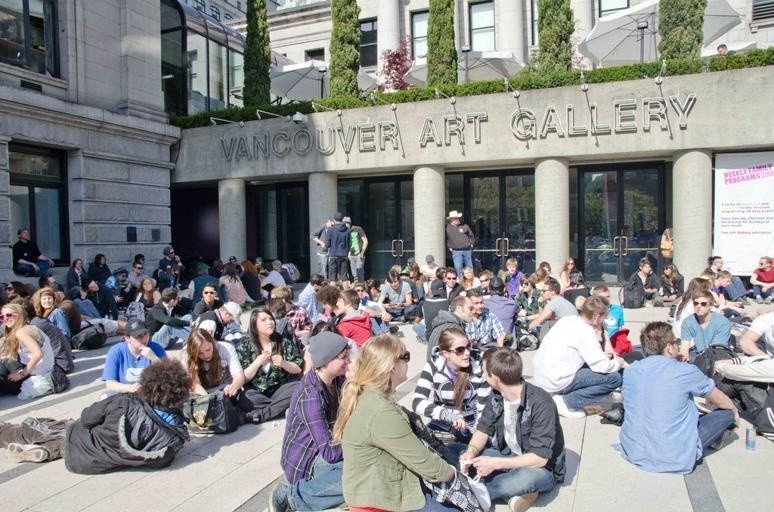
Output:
[692,343,739,378]
[617,280,645,309]
[71,320,107,350]
[123,292,146,322]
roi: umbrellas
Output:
[400,45,528,88]
[576,0,746,67]
[263,57,377,101]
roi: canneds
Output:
[745,428,756,450]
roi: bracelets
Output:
[468,444,481,456]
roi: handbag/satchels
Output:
[181,390,240,434]
[422,459,492,512]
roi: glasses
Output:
[399,351,411,362]
[447,277,457,280]
[520,283,529,285]
[0,312,15,321]
[203,291,215,294]
[693,302,707,307]
[445,343,471,354]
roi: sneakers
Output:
[552,394,586,420]
[268,481,287,512]
[504,489,540,512]
[4,442,49,463]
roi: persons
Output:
[444,210,476,277]
[660,227,674,264]
[341,216,368,283]
[412,326,491,447]
[459,345,568,512]
[324,212,349,283]
[332,332,465,512]
[716,43,736,56]
[11,225,55,277]
[267,330,354,512]
[471,214,660,281]
[309,215,338,283]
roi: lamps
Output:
[579,69,589,91]
[311,102,342,117]
[655,61,667,84]
[435,87,456,105]
[370,93,397,111]
[505,77,521,99]
[209,115,244,128]
[256,109,291,123]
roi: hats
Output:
[124,317,150,338]
[309,330,349,369]
[446,210,462,220]
